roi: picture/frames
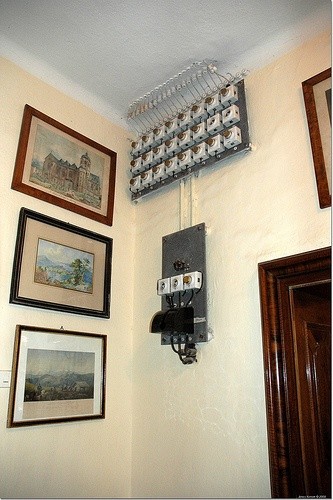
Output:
[301,67,331,209]
[10,104,117,227]
[8,207,114,321]
[5,324,107,428]
